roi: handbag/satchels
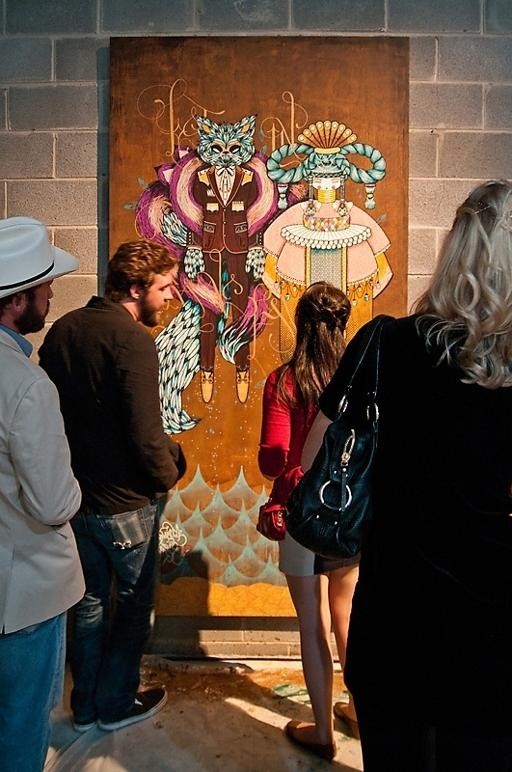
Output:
[256,500,288,543]
[288,312,384,560]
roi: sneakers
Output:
[72,716,97,732]
[98,686,168,733]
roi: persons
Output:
[255,280,362,762]
[0,213,87,772]
[298,178,511,771]
[35,241,189,735]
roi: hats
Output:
[1,216,78,297]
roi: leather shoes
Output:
[334,701,360,740]
[286,719,336,759]
